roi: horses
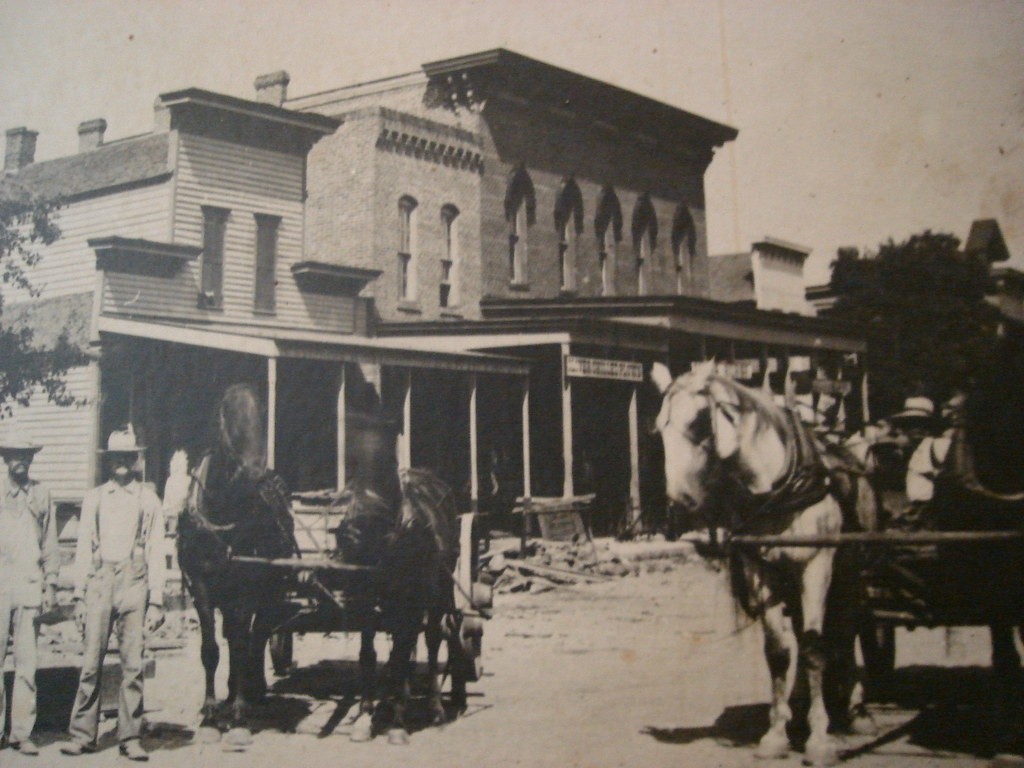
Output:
[336,417,460,738]
[644,291,1023,760]
[176,376,298,730]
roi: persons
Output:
[0,431,50,754]
[56,431,169,762]
[884,396,989,521]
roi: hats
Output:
[891,396,934,420]
[0,434,43,454]
[96,430,147,453]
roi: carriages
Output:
[175,363,494,736]
[649,266,1024,768]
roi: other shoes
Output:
[61,739,95,755]
[12,739,39,756]
[120,739,148,760]
